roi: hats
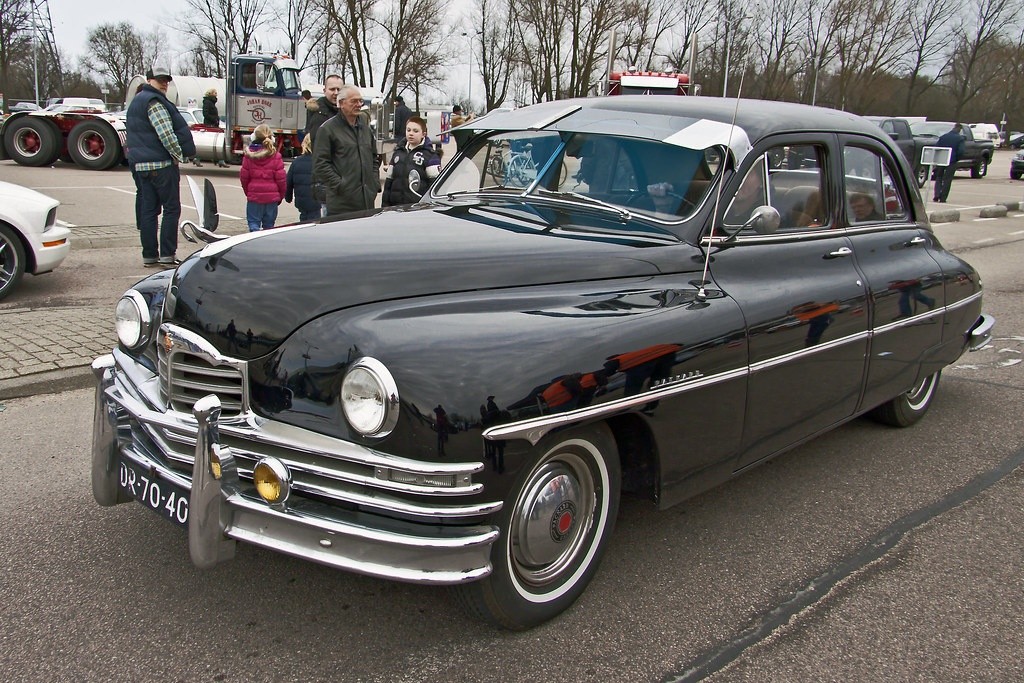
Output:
[145,66,172,81]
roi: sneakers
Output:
[143,258,162,267]
[160,256,183,268]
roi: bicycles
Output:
[486,143,568,189]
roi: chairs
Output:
[779,186,823,228]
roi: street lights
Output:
[461,31,483,114]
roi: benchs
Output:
[631,180,883,229]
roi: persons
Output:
[192,88,230,168]
[932,123,964,203]
[572,139,639,193]
[125,68,197,270]
[240,124,286,232]
[223,318,255,359]
[389,393,504,463]
[451,105,472,128]
[301,90,313,133]
[311,84,382,216]
[886,280,936,322]
[381,116,442,207]
[647,153,789,228]
[790,300,840,347]
[541,343,685,418]
[395,96,412,144]
[848,195,883,222]
[286,134,313,223]
[306,75,345,221]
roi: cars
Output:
[1009,148,1024,179]
[91,96,996,631]
[767,145,817,170]
[968,124,1024,149]
[0,181,71,303]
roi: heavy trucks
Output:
[0,33,397,171]
[593,29,702,96]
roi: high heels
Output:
[218,161,230,168]
[193,159,203,167]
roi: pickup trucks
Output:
[909,122,994,179]
[842,116,940,189]
